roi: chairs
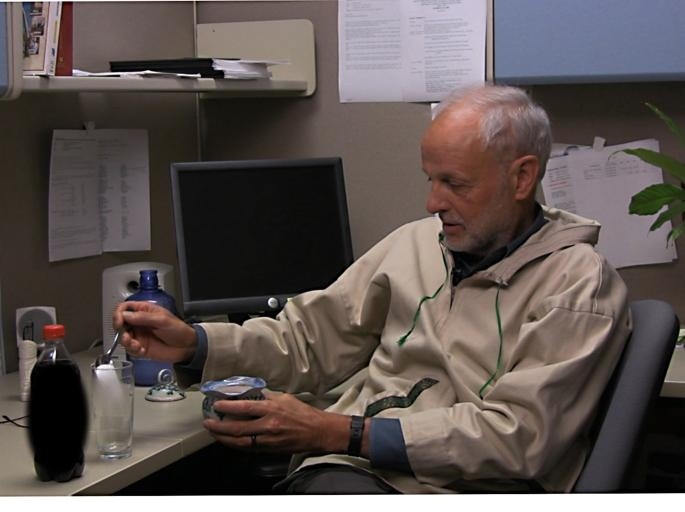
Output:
[571,299,681,492]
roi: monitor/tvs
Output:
[169,156,355,316]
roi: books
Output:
[21,2,62,76]
[108,57,280,79]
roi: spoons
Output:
[94,308,131,366]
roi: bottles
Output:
[127,270,184,387]
[26,326,87,481]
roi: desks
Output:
[659,329,685,399]
[1,345,369,495]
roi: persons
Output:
[113,84,634,494]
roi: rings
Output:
[251,434,256,449]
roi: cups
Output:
[89,358,135,461]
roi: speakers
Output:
[101,260,179,360]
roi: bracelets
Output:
[347,415,364,457]
